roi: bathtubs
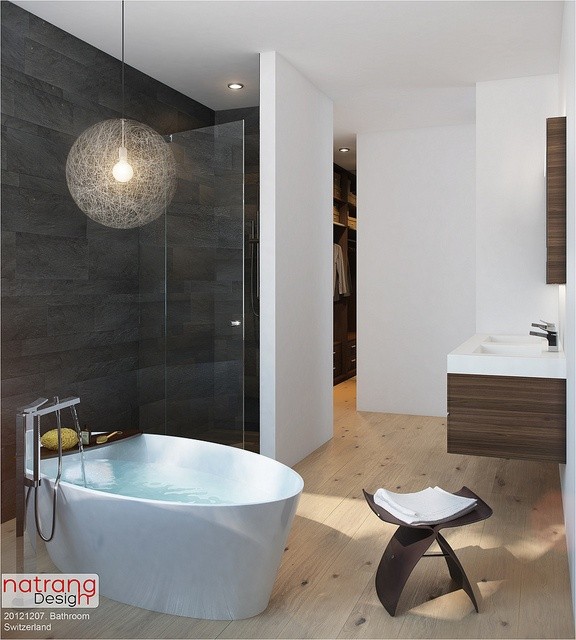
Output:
[38,430,305,621]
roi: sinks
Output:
[477,338,542,357]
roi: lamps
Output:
[65,0,175,230]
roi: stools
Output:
[361,487,492,618]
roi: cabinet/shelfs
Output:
[333,162,355,385]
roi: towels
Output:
[374,484,476,525]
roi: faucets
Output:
[16,395,83,480]
[530,319,559,333]
[528,329,559,348]
